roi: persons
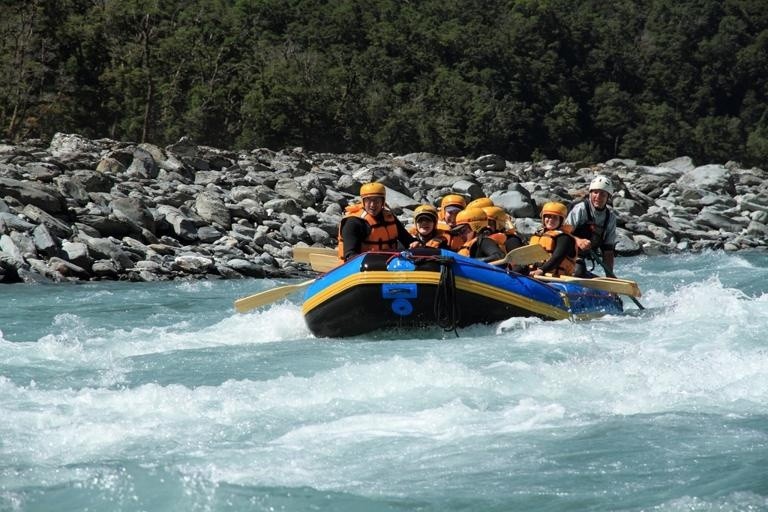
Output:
[407,175,618,277]
[336,183,426,264]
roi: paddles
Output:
[310,254,339,272]
[235,278,315,313]
[543,272,641,297]
[294,247,339,264]
[534,275,634,295]
[488,244,550,266]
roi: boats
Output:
[302,246,625,339]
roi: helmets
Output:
[456,208,488,233]
[542,202,567,221]
[360,183,386,208]
[466,198,494,208]
[414,205,438,226]
[589,177,613,195]
[441,195,466,220]
[481,207,506,231]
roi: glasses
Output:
[456,227,467,236]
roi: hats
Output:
[445,206,461,211]
[450,225,464,231]
[417,214,435,221]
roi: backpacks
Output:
[566,197,610,230]
